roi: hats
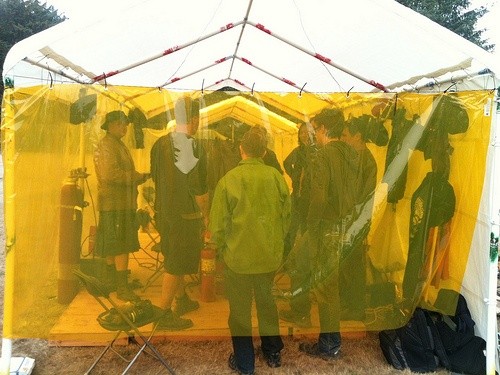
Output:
[101,111,128,130]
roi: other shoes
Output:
[279,309,311,328]
[175,301,199,314]
[299,341,342,360]
[261,344,281,367]
[229,353,255,375]
[154,315,193,330]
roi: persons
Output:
[152,98,207,328]
[207,129,293,375]
[279,108,360,357]
[250,118,315,264]
[93,111,151,302]
[341,119,377,320]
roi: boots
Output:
[106,265,135,301]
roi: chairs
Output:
[72,268,176,375]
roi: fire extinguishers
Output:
[201,241,217,302]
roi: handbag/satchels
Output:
[380,290,486,375]
